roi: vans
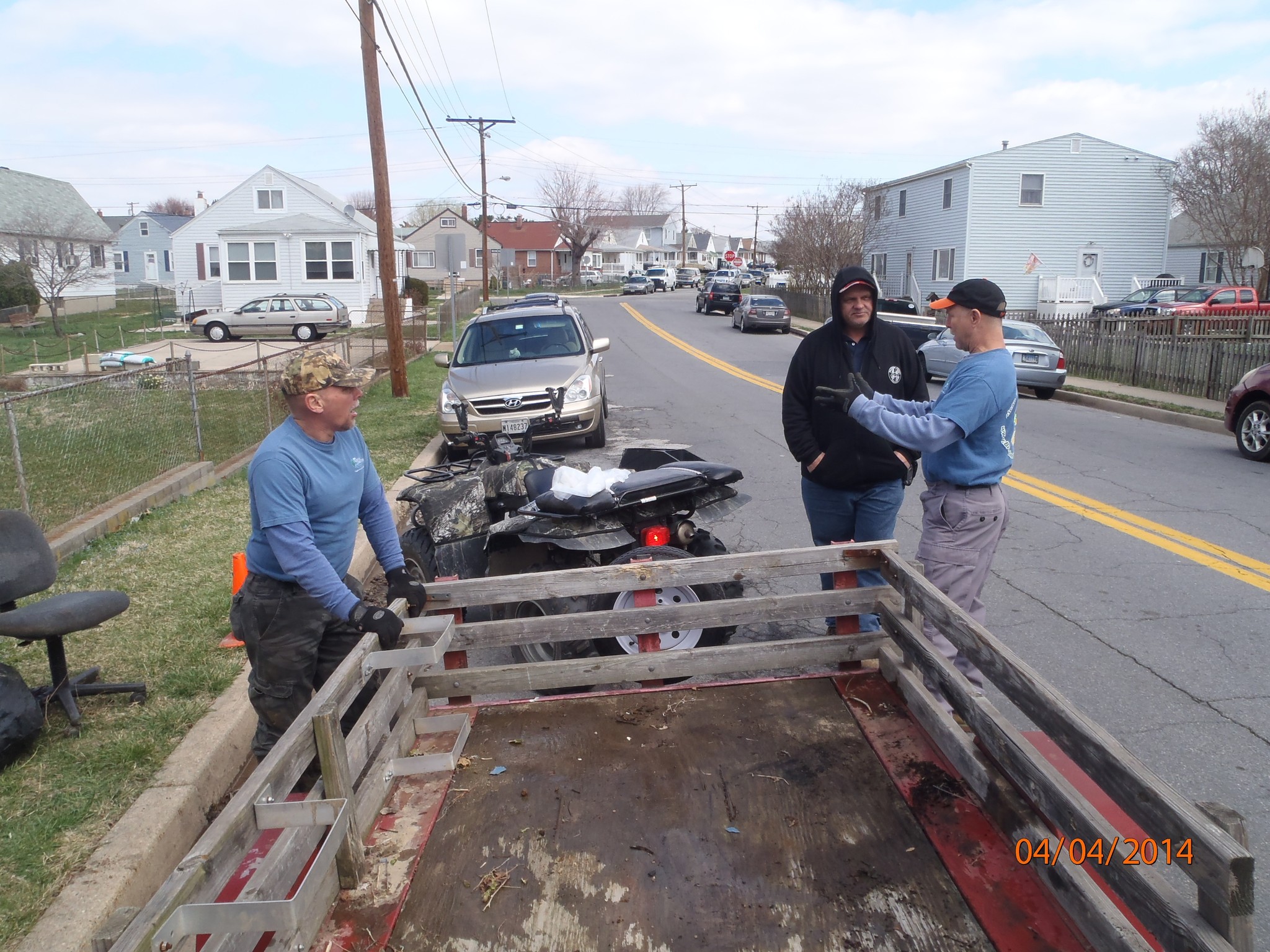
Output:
[645,267,676,292]
[711,269,743,289]
[676,267,701,288]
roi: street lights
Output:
[482,176,511,301]
[753,230,770,269]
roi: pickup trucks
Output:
[824,296,946,351]
[1129,287,1270,343]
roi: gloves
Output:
[349,603,404,651]
[386,567,427,617]
[852,372,874,402]
[815,372,862,413]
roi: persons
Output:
[229,348,427,792]
[782,266,930,635]
[814,278,1018,727]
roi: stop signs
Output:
[733,257,742,266]
[725,251,735,261]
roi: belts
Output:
[952,483,992,490]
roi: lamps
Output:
[283,233,292,239]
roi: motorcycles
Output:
[396,388,744,696]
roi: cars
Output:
[704,272,716,285]
[916,320,1066,399]
[732,295,791,334]
[615,269,655,294]
[738,262,777,288]
[1224,362,1270,461]
[1093,285,1204,337]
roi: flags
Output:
[1024,252,1043,275]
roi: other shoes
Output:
[824,626,836,637]
[950,710,974,733]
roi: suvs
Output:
[696,280,742,315]
[556,270,604,286]
[437,292,610,462]
[189,293,351,342]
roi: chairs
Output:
[0,510,146,734]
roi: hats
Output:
[840,278,874,294]
[929,278,1006,319]
[280,349,377,395]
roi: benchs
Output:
[9,311,45,337]
